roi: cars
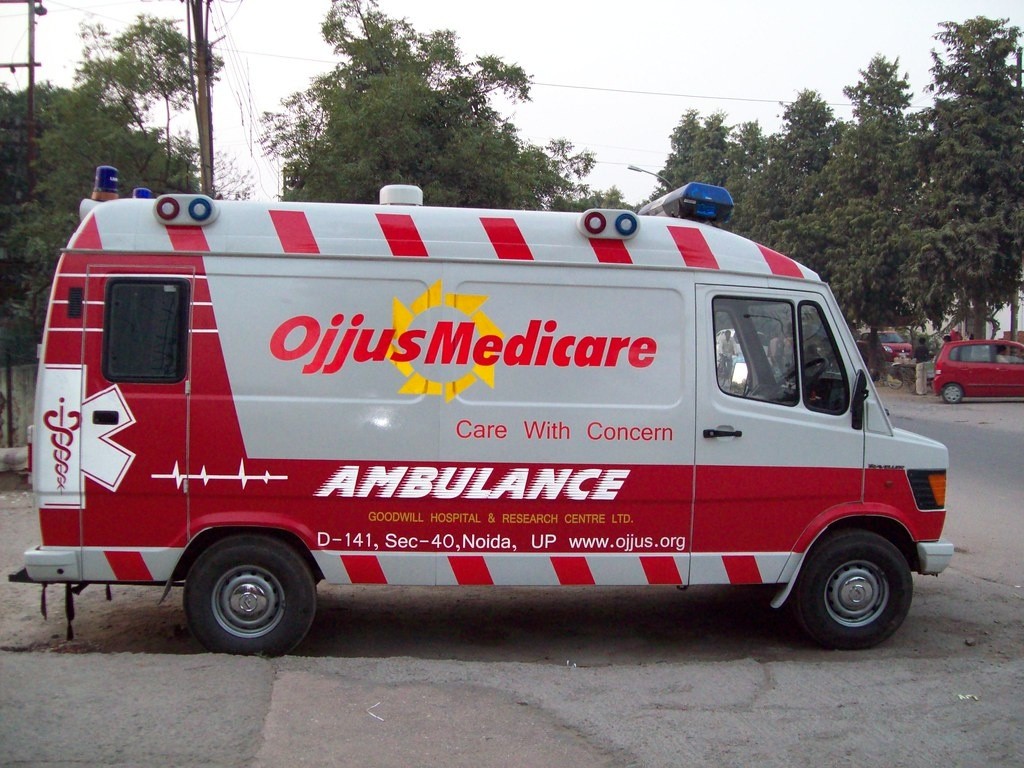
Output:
[934,339,1024,405]
[855,331,912,362]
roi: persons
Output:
[914,337,929,395]
[767,333,824,378]
[996,346,1009,364]
[718,330,738,372]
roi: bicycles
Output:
[885,363,916,393]
[716,352,739,389]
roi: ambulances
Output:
[8,164,956,660]
